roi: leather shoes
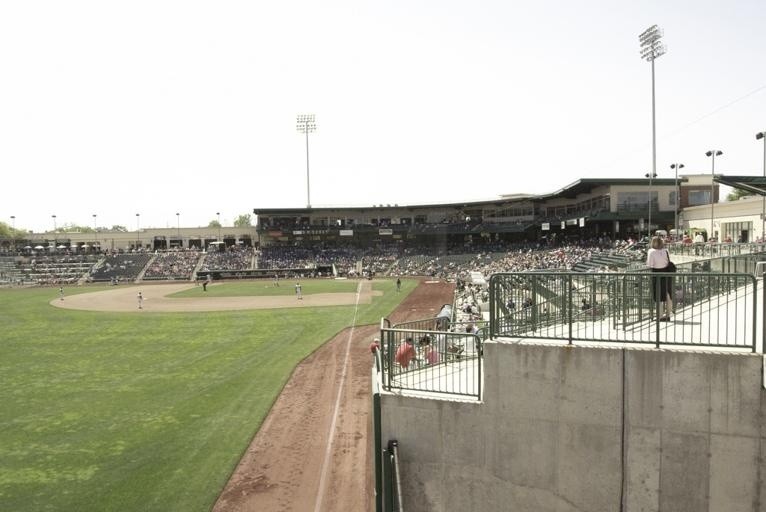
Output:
[660,317,670,322]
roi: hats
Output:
[374,338,380,341]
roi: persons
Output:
[295,283,303,300]
[137,291,144,309]
[273,273,280,286]
[448,201,766,346]
[201,280,210,292]
[60,286,65,300]
[419,337,439,366]
[396,277,402,292]
[1,203,446,287]
[396,337,418,368]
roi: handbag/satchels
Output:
[668,261,676,272]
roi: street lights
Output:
[670,164,684,243]
[707,150,723,243]
[296,114,316,208]
[645,173,658,236]
[756,131,765,242]
[638,24,665,179]
[11,212,220,248]
